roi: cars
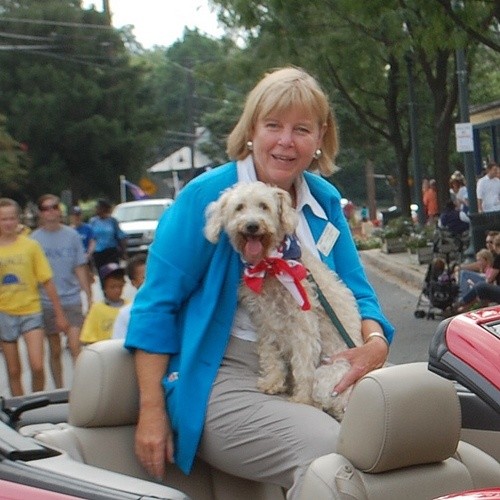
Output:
[111,198,174,260]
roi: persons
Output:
[133,65,392,490]
[421,163,500,317]
[1,192,145,398]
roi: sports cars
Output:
[0,304,500,500]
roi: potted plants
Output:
[417,237,434,264]
[375,217,413,254]
[404,236,417,257]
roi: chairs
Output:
[34,336,285,500]
[295,359,500,500]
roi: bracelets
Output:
[363,332,389,351]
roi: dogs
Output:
[201,178,386,423]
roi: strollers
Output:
[414,257,462,321]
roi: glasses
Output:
[39,204,58,211]
[486,241,492,243]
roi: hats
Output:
[69,206,81,215]
[100,264,126,280]
[98,201,112,210]
